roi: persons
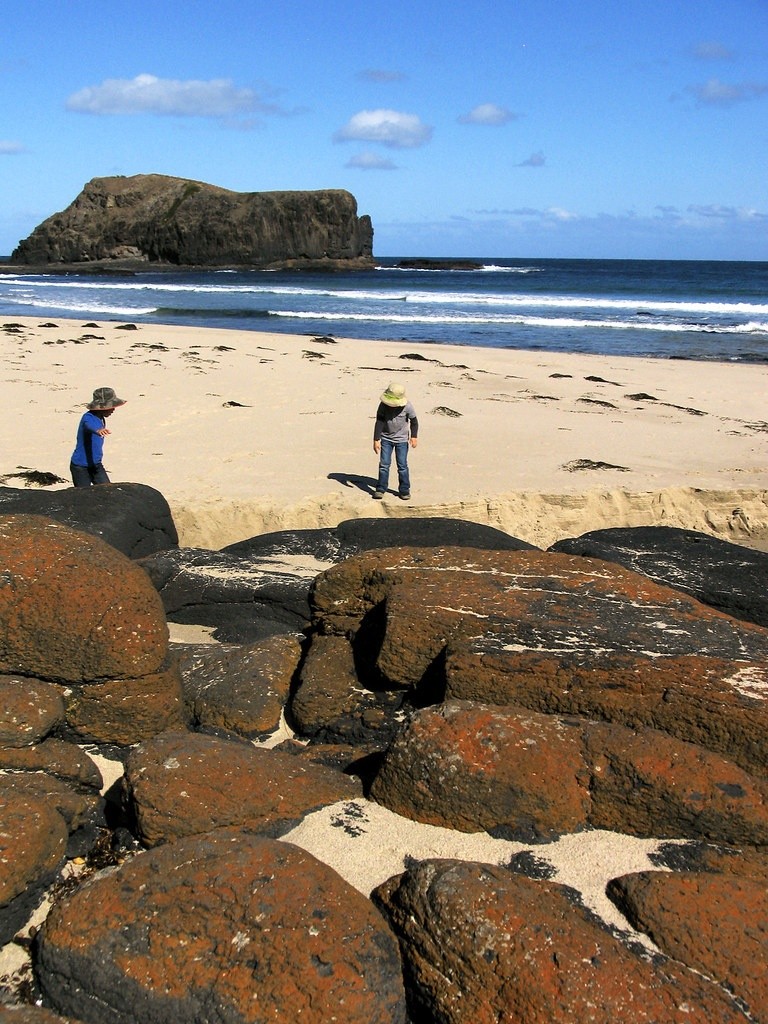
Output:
[373,383,418,500]
[70,387,128,487]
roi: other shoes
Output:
[399,492,410,500]
[373,491,384,499]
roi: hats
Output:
[379,384,408,407]
[86,387,127,410]
